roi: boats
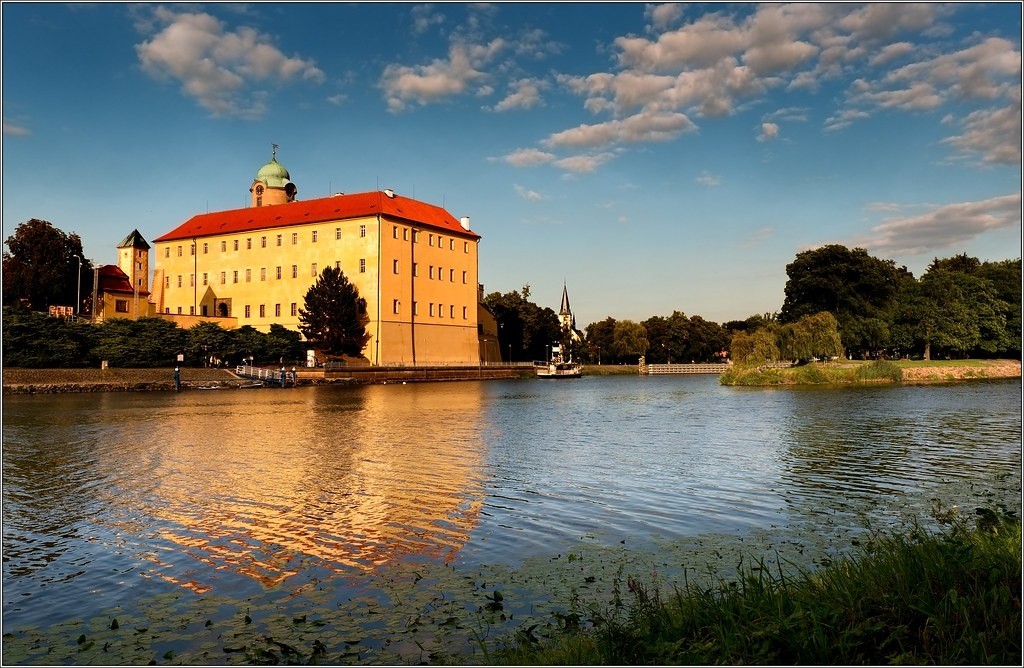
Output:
[536,349,583,378]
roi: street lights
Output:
[73,254,81,314]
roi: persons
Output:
[174,365,181,390]
[279,356,284,368]
